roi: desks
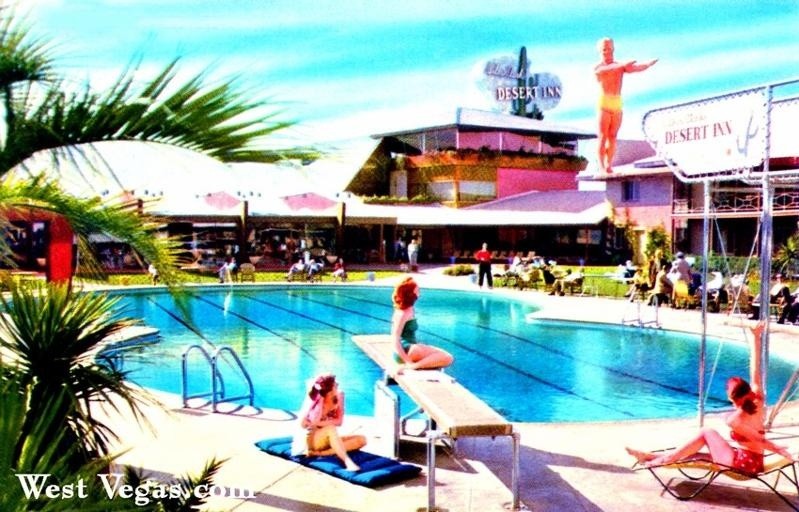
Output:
[583,273,633,297]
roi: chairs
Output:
[565,275,586,295]
[632,437,799,511]
[223,263,257,281]
[726,289,743,312]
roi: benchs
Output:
[354,333,523,511]
[292,271,323,283]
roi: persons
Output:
[285,259,305,282]
[193,254,237,283]
[621,239,799,325]
[148,264,159,285]
[300,375,367,472]
[306,256,322,278]
[594,37,659,173]
[407,239,419,272]
[332,263,347,283]
[625,322,799,474]
[496,255,585,296]
[474,242,493,289]
[391,276,454,375]
